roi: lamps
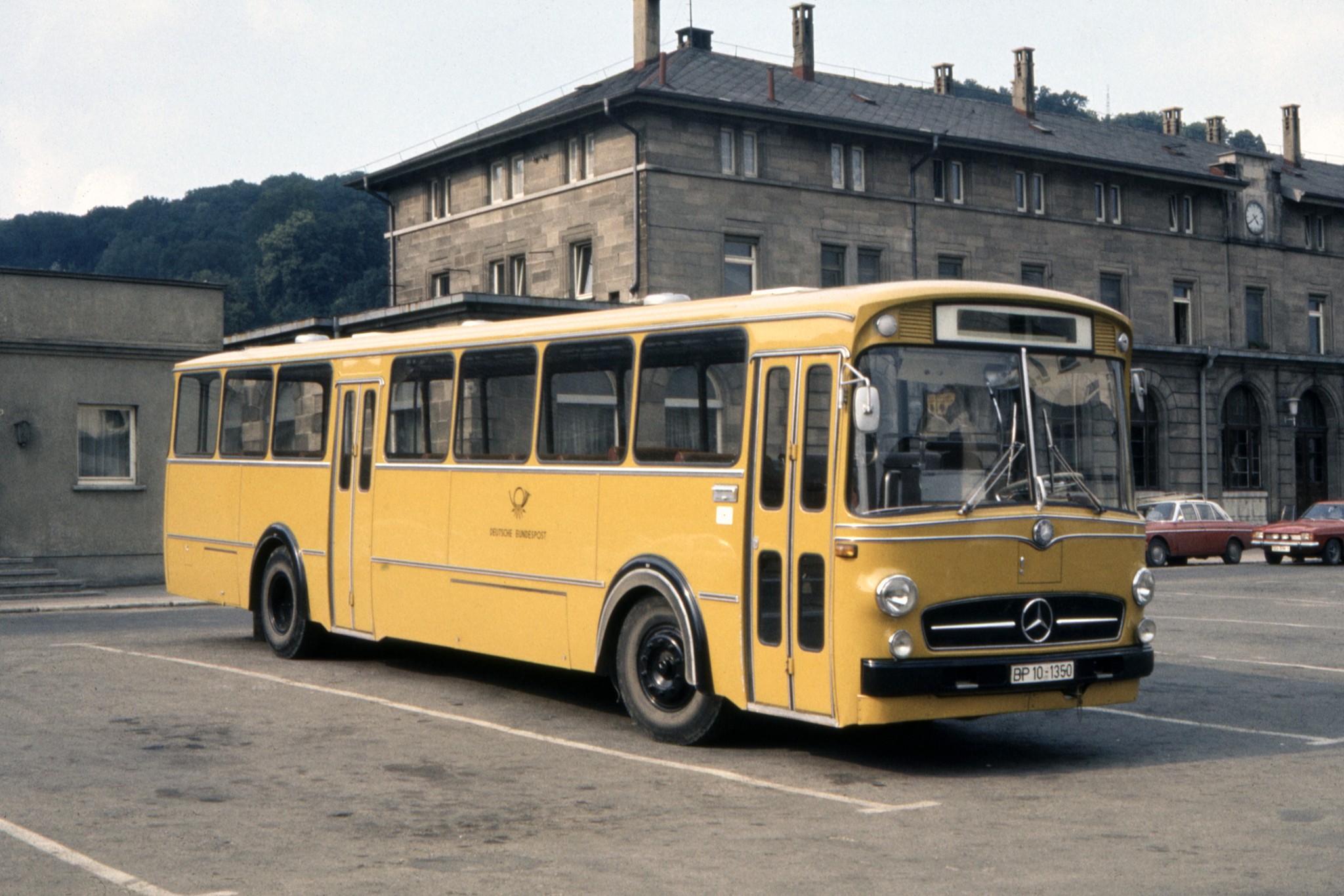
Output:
[1285,397,1300,428]
[13,418,32,450]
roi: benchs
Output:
[611,447,938,504]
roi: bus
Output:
[162,278,1156,748]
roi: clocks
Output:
[1245,200,1266,237]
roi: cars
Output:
[1250,500,1344,566]
[1135,499,1257,568]
